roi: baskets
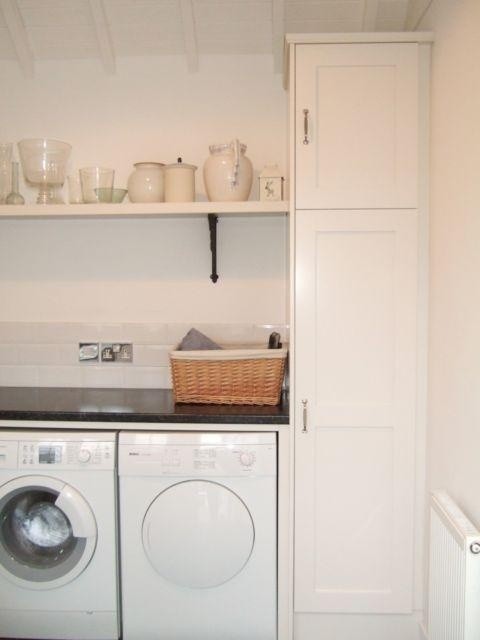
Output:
[167,340,289,408]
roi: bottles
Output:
[0,142,15,205]
[164,157,197,203]
[127,162,166,205]
[5,161,25,204]
[257,163,284,201]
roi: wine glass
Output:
[17,138,72,205]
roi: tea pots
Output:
[202,138,254,202]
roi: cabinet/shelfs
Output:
[284,31,433,209]
[289,209,432,636]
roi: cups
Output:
[80,166,116,203]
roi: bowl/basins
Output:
[93,187,128,203]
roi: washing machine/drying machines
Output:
[0,431,122,640]
[117,430,278,640]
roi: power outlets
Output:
[100,343,133,363]
